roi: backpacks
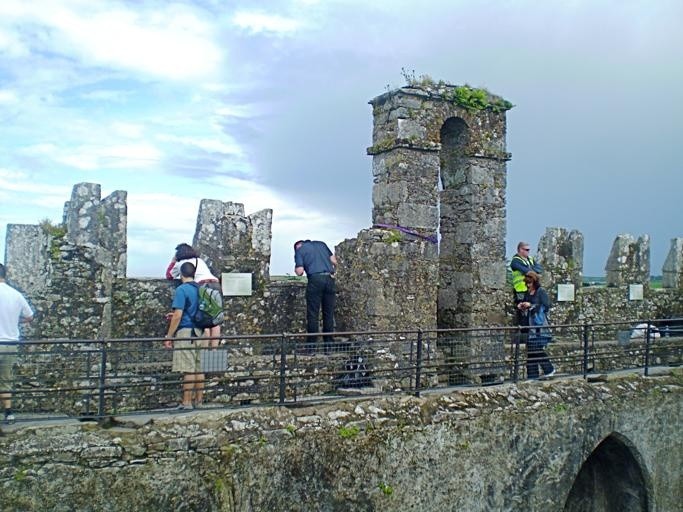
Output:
[184,281,224,329]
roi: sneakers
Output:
[174,403,204,411]
[296,348,317,356]
[323,348,340,355]
[542,370,557,378]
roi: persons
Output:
[515,271,555,381]
[165,243,224,352]
[0,263,35,425]
[292,239,337,356]
[508,240,544,343]
[162,261,207,413]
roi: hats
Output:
[525,271,541,283]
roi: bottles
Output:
[199,300,206,311]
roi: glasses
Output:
[522,249,531,252]
